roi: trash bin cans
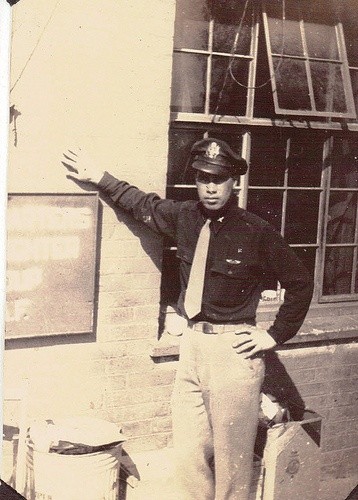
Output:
[27,417,126,500]
[251,407,325,500]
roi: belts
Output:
[188,320,240,334]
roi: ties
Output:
[184,219,211,319]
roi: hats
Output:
[190,138,248,177]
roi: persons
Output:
[60,135,316,500]
